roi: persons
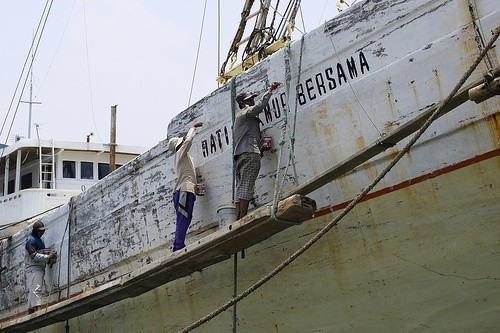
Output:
[167,121,206,253]
[24,220,58,315]
[233,81,282,221]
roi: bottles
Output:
[196,183,206,196]
[262,137,272,150]
[43,249,49,255]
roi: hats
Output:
[235,92,258,103]
[168,136,183,154]
[33,221,48,231]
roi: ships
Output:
[0,0,500,333]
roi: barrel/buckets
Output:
[216,204,237,230]
